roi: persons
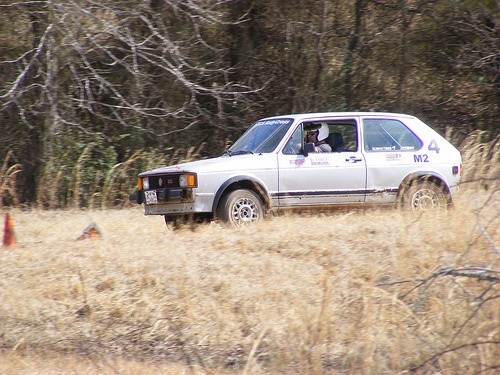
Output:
[290,122,332,153]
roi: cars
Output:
[137,111,464,230]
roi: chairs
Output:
[325,132,343,152]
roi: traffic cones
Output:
[77,223,101,240]
[3,212,17,248]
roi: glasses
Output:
[308,132,315,135]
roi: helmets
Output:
[304,122,329,143]
